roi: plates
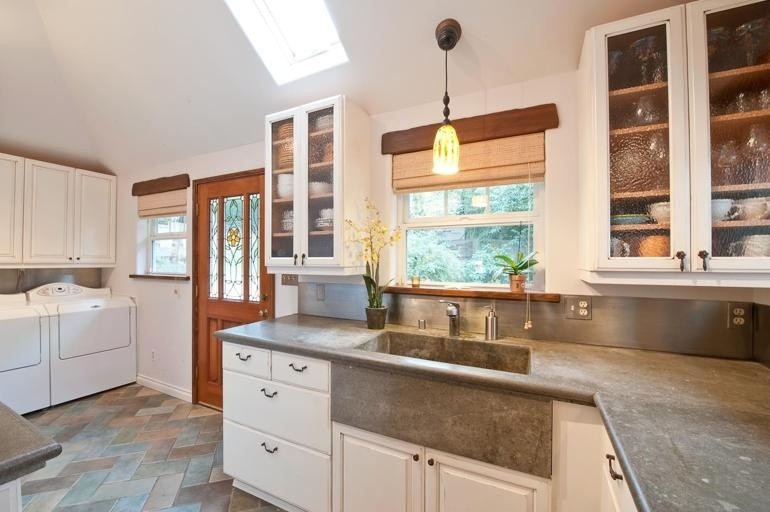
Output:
[277,122,295,139]
[277,144,293,167]
[609,213,652,225]
[313,113,333,130]
[313,218,334,230]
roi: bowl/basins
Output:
[308,179,333,193]
[276,174,294,196]
[638,234,670,257]
[647,200,669,226]
[317,207,334,218]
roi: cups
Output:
[711,196,743,223]
[739,197,770,220]
[729,234,770,258]
[610,237,630,257]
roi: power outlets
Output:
[726,301,751,329]
[564,295,592,321]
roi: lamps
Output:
[433,16,461,175]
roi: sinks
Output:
[354,331,531,377]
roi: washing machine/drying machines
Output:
[27,282,138,408]
[0,291,50,417]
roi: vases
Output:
[365,299,388,330]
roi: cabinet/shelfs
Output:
[265,94,385,277]
[0,152,25,269]
[331,418,551,512]
[222,342,331,512]
[23,158,118,269]
[551,400,603,511]
[577,0,770,290]
[603,428,638,511]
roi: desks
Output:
[0,399,64,512]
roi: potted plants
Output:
[407,252,425,288]
[494,250,541,293]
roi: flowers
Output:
[345,194,404,306]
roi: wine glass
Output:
[708,17,767,72]
[604,34,668,90]
[717,122,769,186]
[646,130,670,189]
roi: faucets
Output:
[439,300,461,337]
